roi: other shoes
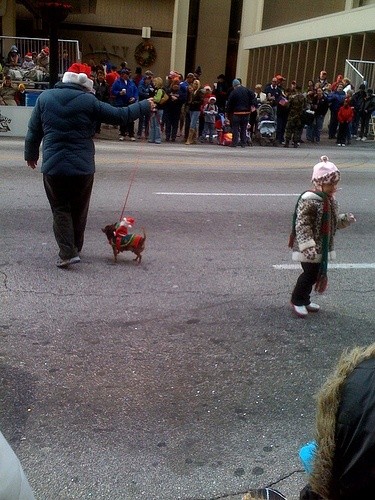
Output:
[290,301,320,317]
[350,134,367,141]
[131,137,135,141]
[54,256,80,267]
[337,143,346,146]
[280,141,285,145]
[137,135,141,138]
[229,142,245,148]
[119,135,125,140]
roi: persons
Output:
[288,155,357,315]
[0,45,375,148]
[24,63,156,267]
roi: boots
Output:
[294,141,297,148]
[185,128,201,145]
[284,139,289,148]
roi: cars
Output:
[218,123,239,145]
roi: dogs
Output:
[101,223,146,266]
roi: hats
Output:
[121,68,130,74]
[201,83,217,103]
[276,74,283,80]
[10,45,18,52]
[344,96,350,103]
[187,73,196,78]
[310,155,340,182]
[359,83,365,89]
[25,52,32,58]
[232,79,240,87]
[41,46,49,55]
[62,62,93,92]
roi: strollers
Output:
[256,103,279,148]
